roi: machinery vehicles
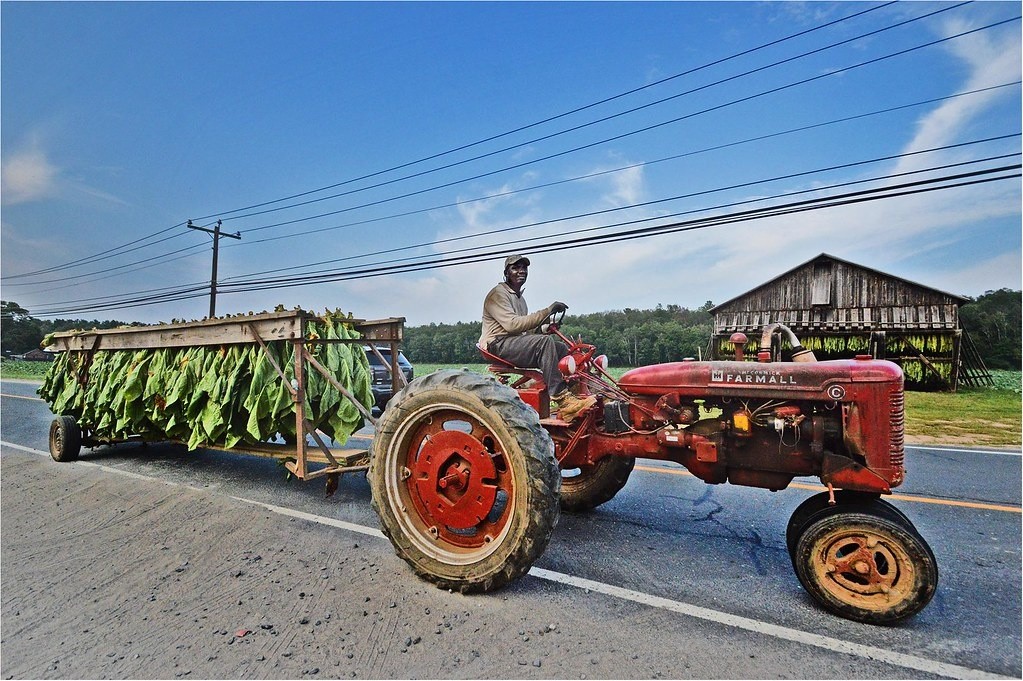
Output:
[44,306,939,628]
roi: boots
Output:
[550,388,597,423]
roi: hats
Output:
[505,255,530,269]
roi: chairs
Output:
[477,342,518,368]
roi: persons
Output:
[480,255,574,402]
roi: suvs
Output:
[362,345,414,412]
